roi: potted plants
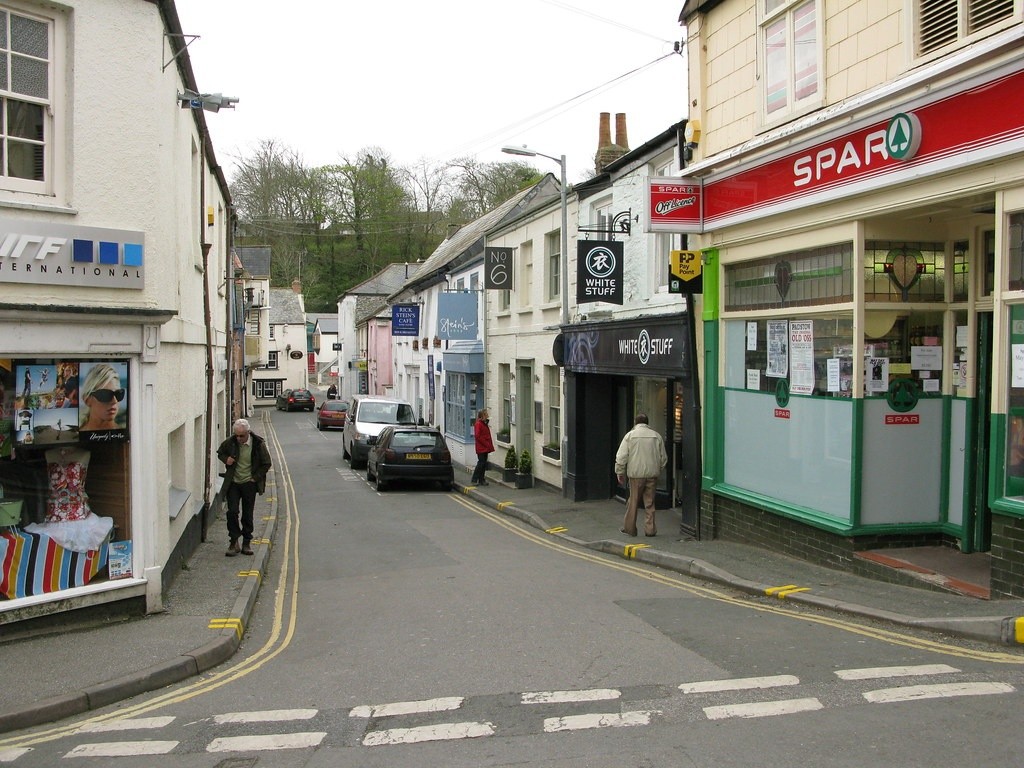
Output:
[502,445,518,482]
[514,449,532,489]
[496,427,510,443]
[541,441,560,460]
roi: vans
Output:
[341,394,425,471]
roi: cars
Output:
[315,400,351,431]
[366,423,455,493]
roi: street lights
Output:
[501,143,571,486]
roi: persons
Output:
[470,407,495,486]
[615,412,668,536]
[327,384,337,399]
[79,363,125,431]
[21,365,76,409]
[218,418,272,556]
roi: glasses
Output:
[90,388,125,403]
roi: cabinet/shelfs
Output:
[745,315,908,364]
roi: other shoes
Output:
[644,533,655,537]
[619,526,631,535]
[471,481,480,486]
[478,480,489,485]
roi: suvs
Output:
[275,388,315,412]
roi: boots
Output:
[224,538,240,556]
[241,538,254,555]
